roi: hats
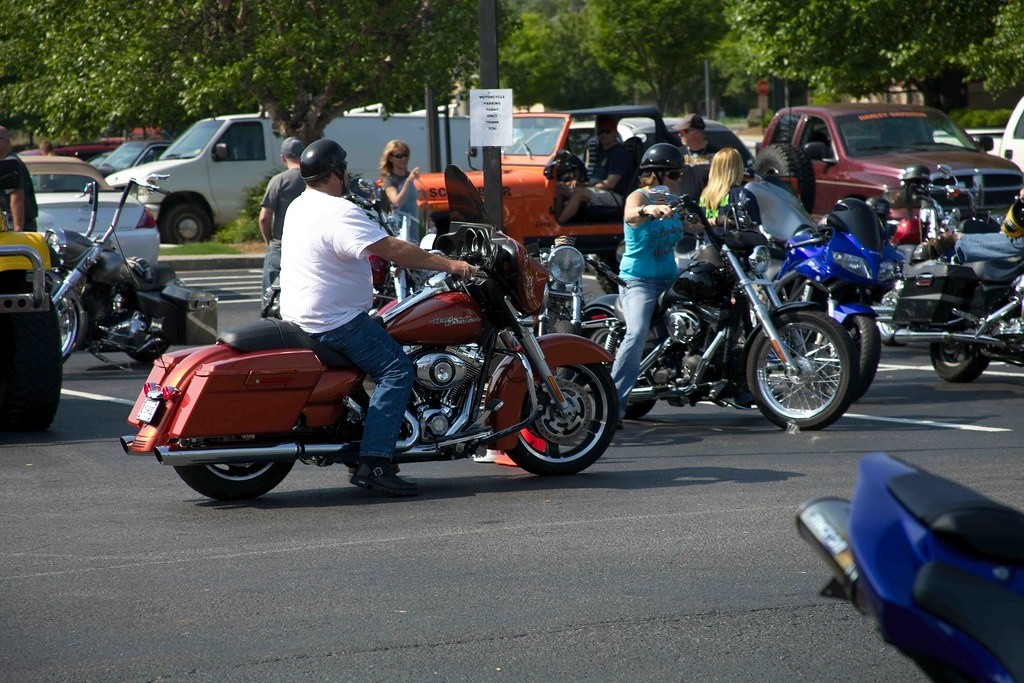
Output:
[280,136,306,157]
[598,120,617,130]
[672,114,706,132]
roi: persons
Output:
[280,138,477,496]
[379,140,420,302]
[928,185,1024,265]
[259,136,305,306]
[555,119,634,224]
[0,126,38,282]
[672,113,721,204]
[698,146,769,239]
[611,144,736,429]
[40,141,65,188]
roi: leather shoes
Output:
[351,459,417,493]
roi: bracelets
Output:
[715,216,723,227]
[638,204,649,217]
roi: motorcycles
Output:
[865,160,1024,381]
[118,178,621,502]
[45,173,194,365]
[769,196,908,399]
[512,234,630,380]
[577,193,861,432]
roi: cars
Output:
[17,125,173,178]
[933,95,1024,174]
[11,156,160,264]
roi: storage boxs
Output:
[169,348,323,435]
[127,347,194,426]
[894,262,980,327]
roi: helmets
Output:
[1001,198,1024,238]
[298,138,346,181]
[901,164,931,185]
[865,196,890,216]
[640,143,686,171]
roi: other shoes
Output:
[617,417,624,430]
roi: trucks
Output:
[106,107,305,246]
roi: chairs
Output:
[624,136,645,160]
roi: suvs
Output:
[755,101,1024,225]
[368,103,755,267]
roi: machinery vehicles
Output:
[0,207,63,434]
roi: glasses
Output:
[681,127,696,134]
[389,152,409,159]
[658,169,684,182]
[599,127,612,135]
